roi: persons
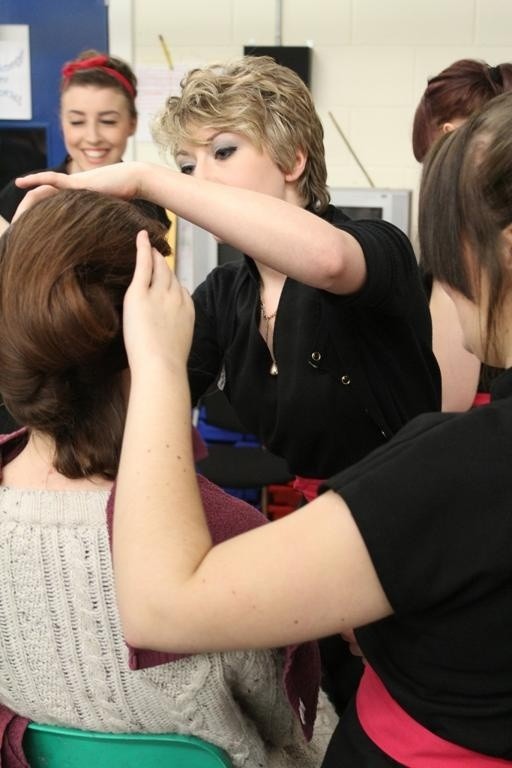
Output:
[1,49,171,276]
[107,84,510,767]
[0,182,333,768]
[402,57,510,413]
[2,53,446,684]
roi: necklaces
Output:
[258,306,277,343]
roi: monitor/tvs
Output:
[173,187,413,298]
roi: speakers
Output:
[0,125,47,224]
[243,45,309,90]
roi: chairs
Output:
[22,724,234,768]
